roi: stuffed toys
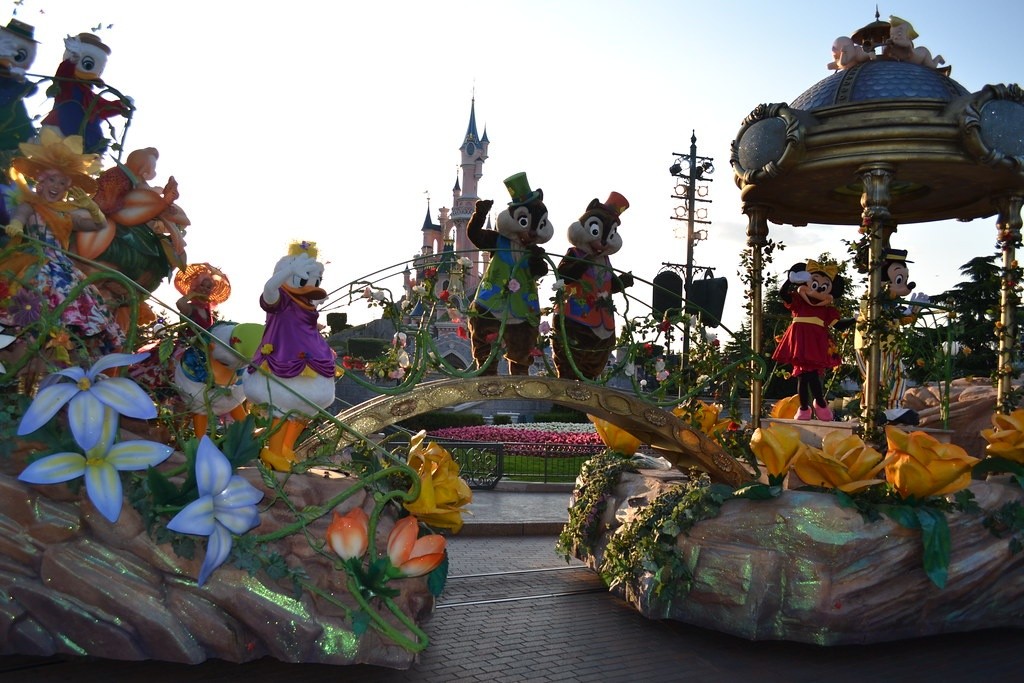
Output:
[467,171,554,376]
[243,241,345,419]
[854,250,930,416]
[41,32,135,159]
[772,259,841,422]
[553,191,634,382]
[0,19,41,185]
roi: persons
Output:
[175,323,267,440]
[174,262,231,436]
[128,145,188,238]
[7,128,127,351]
